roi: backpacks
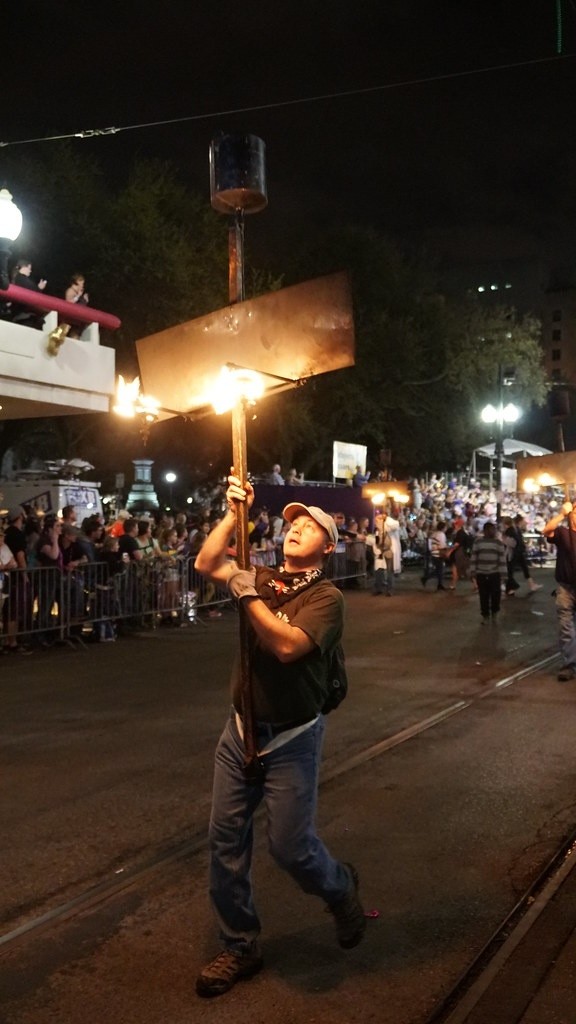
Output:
[295,583,348,715]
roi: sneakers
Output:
[197,930,263,996]
[323,863,365,949]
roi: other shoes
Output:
[559,666,576,680]
[3,644,20,652]
[421,577,428,585]
[438,584,446,590]
[375,592,381,595]
[386,593,392,597]
[481,614,499,625]
[209,611,222,617]
[450,586,455,590]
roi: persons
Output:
[470,523,504,627]
[541,501,576,681]
[188,463,358,626]
[345,466,576,596]
[0,505,192,656]
[193,467,366,998]
[0,258,89,340]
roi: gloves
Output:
[226,563,262,606]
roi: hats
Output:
[283,502,338,560]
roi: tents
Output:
[473,438,556,468]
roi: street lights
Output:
[481,402,518,523]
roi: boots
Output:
[507,589,516,595]
[527,577,543,593]
